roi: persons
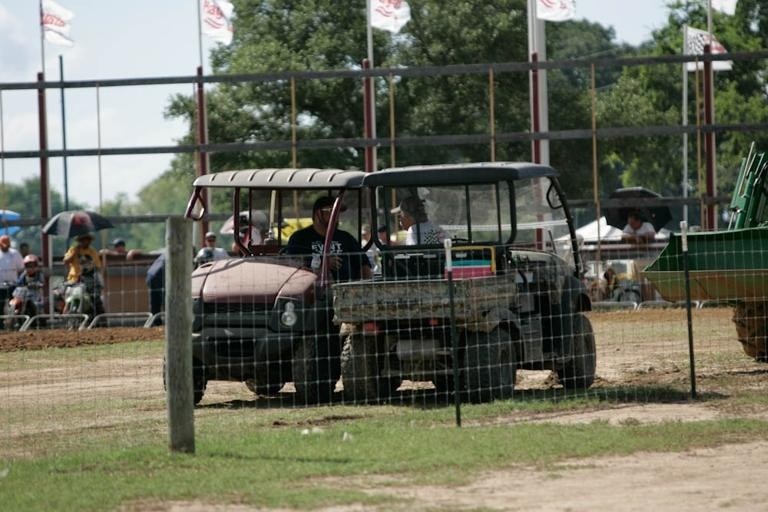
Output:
[373,225,400,274]
[360,223,377,274]
[621,210,658,301]
[0,232,232,331]
[286,196,375,283]
[389,196,452,257]
[230,215,265,257]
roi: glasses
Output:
[320,207,333,213]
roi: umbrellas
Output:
[272,217,315,246]
[0,209,24,236]
[601,185,673,233]
[220,209,269,235]
[40,210,116,238]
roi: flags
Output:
[535,1,577,22]
[42,1,76,45]
[370,0,410,34]
[686,25,735,73]
[201,0,240,47]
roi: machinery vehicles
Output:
[637,138,768,363]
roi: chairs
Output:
[469,240,505,262]
[247,245,291,256]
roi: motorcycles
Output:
[1,272,107,334]
[580,266,645,303]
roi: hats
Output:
[313,196,348,212]
[205,231,217,237]
[23,230,127,268]
[390,196,426,214]
